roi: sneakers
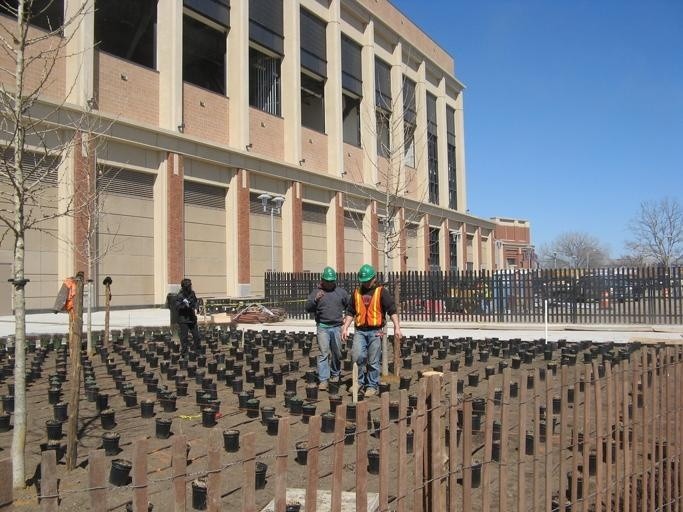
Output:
[365,387,379,396]
[349,383,362,393]
[329,376,339,383]
[319,382,329,392]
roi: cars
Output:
[541,272,683,303]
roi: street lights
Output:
[553,248,557,270]
[450,232,462,272]
[527,247,535,270]
[257,193,286,272]
[495,240,504,271]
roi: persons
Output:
[341,265,401,397]
[305,267,351,391]
[174,279,202,358]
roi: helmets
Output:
[358,264,377,283]
[321,267,337,281]
